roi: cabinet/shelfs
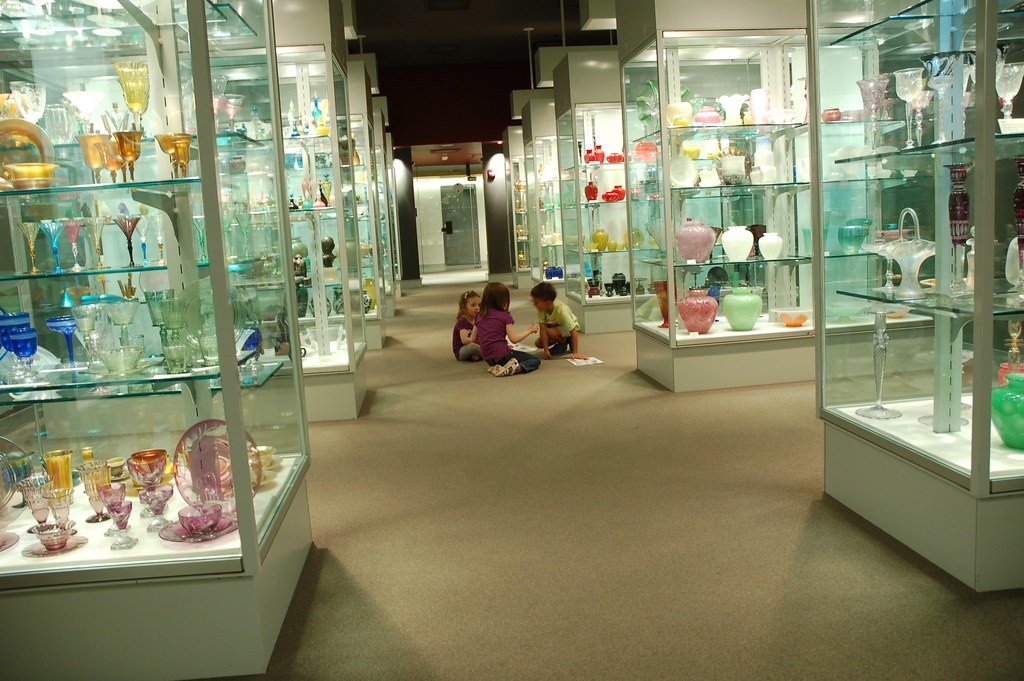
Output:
[0,0,403,680]
[504,0,1024,593]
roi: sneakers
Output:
[488,364,502,375]
[496,358,519,376]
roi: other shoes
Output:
[549,341,567,356]
[568,336,573,351]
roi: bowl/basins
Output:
[31,521,76,551]
[4,163,59,190]
[584,243,599,250]
[770,302,911,327]
[178,502,222,535]
[616,242,626,250]
[95,347,143,373]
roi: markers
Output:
[532,319,538,334]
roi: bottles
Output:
[678,287,762,334]
[648,237,659,249]
[546,266,564,279]
[695,108,720,126]
[991,363,1024,450]
[821,108,841,122]
[677,218,879,262]
[286,150,377,314]
[750,137,776,183]
[612,273,626,295]
[584,145,630,251]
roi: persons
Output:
[452,291,482,362]
[470,281,541,377]
[530,281,588,360]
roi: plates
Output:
[176,280,247,350]
[159,419,281,542]
[0,119,54,180]
[0,436,88,558]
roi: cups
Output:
[722,156,745,185]
[0,81,79,145]
[119,335,144,359]
[162,346,187,373]
[199,337,220,365]
[300,324,348,355]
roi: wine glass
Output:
[856,63,1024,293]
[0,289,186,384]
[193,214,255,263]
[16,215,167,275]
[604,283,615,297]
[77,61,245,184]
[7,450,173,550]
[652,281,676,328]
[700,286,720,322]
